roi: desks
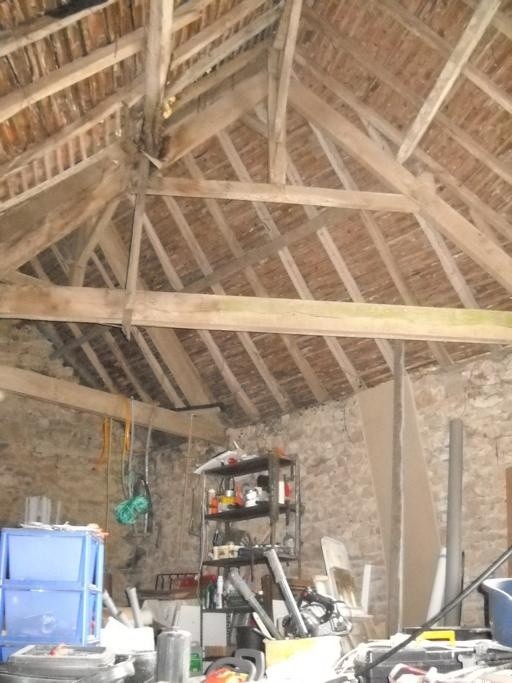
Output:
[137,589,199,632]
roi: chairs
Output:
[155,573,199,592]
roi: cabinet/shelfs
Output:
[200,447,300,650]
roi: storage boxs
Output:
[0,527,104,590]
[262,636,343,678]
[481,578,512,650]
[354,640,512,683]
[0,584,102,664]
